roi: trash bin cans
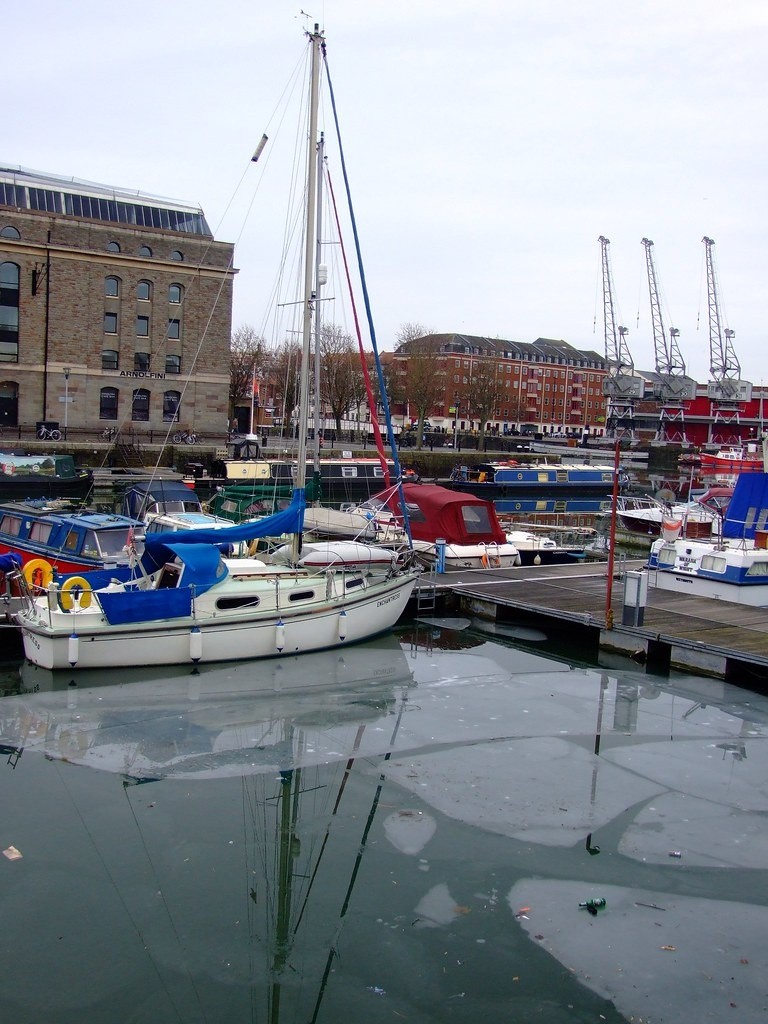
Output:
[36,422,60,440]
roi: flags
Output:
[254,378,258,396]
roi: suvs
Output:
[412,421,432,431]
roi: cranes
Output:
[702,236,752,450]
[641,236,696,450]
[595,235,644,447]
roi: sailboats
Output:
[19,16,423,672]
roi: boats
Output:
[679,466,701,475]
[201,482,378,541]
[698,447,764,468]
[0,497,144,610]
[0,450,418,507]
[503,526,583,560]
[678,453,700,465]
[530,441,650,463]
[108,480,236,546]
[422,460,639,498]
[605,488,736,539]
[361,484,521,569]
[698,465,763,488]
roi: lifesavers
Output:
[249,538,260,555]
[482,553,500,569]
[61,575,92,610]
[22,558,53,590]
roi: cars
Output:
[506,429,583,439]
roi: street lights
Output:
[454,400,460,450]
[61,366,71,439]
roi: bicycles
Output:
[172,429,206,446]
[35,423,62,441]
[96,426,122,444]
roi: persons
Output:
[228,417,239,434]
[102,426,115,440]
